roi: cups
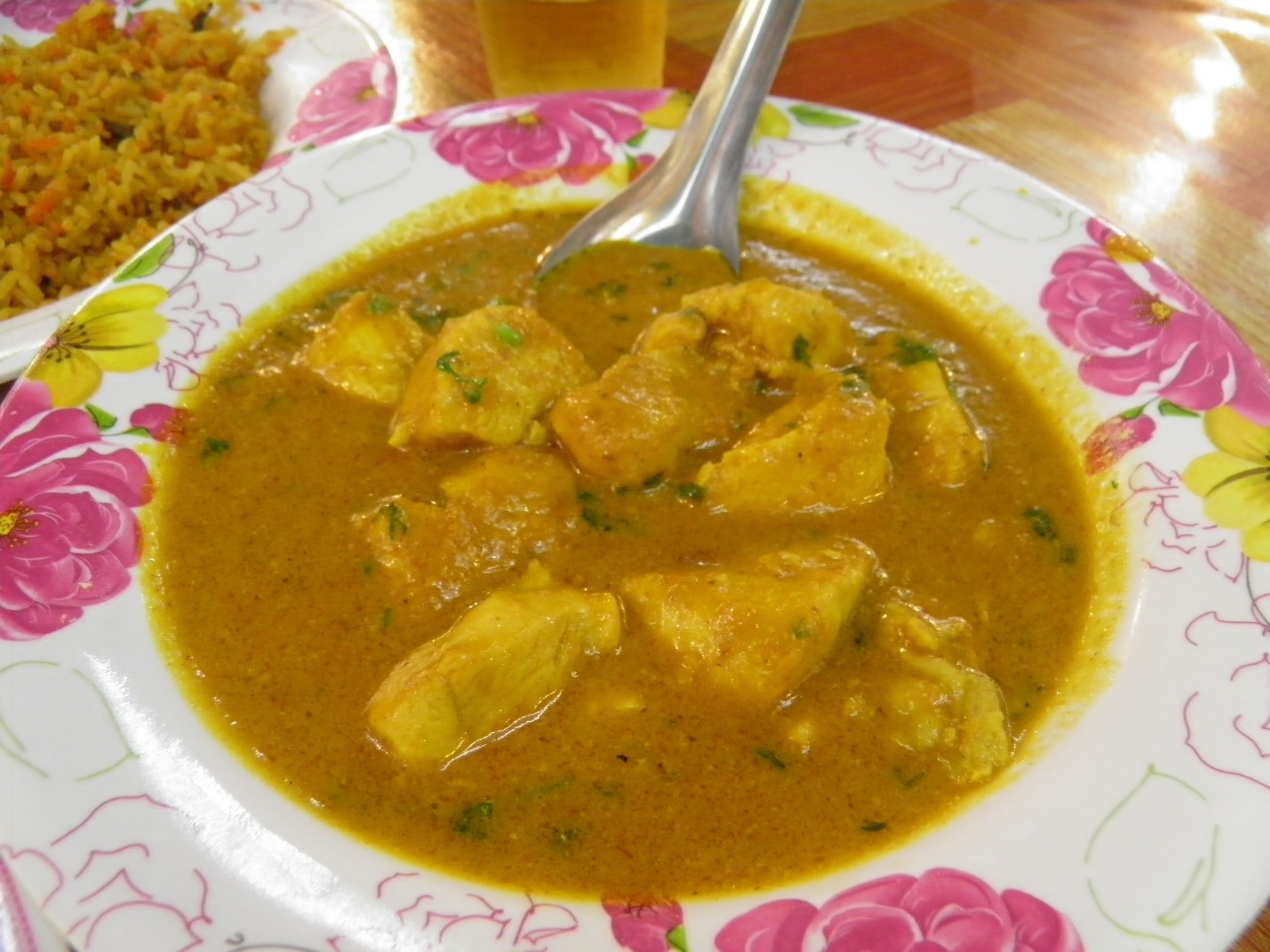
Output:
[473,1,665,97]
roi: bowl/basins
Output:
[2,89,1270,951]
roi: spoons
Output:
[539,0,805,279]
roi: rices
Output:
[0,1,297,323]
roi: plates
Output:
[0,0,397,384]
